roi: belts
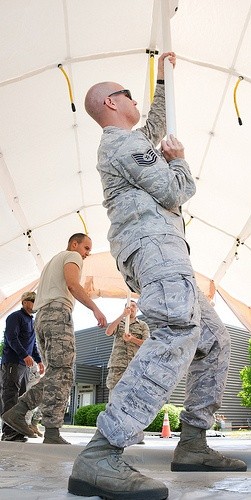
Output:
[41,302,70,311]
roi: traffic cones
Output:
[159,411,172,438]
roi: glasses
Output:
[104,89,132,105]
[22,297,35,304]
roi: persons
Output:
[0,291,51,442]
[0,233,107,445]
[68,50,247,500]
[105,300,150,398]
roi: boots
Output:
[1,401,43,438]
[67,429,168,500]
[42,425,70,444]
[171,419,247,472]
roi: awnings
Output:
[0,0,251,339]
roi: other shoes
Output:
[29,425,43,437]
[29,433,38,438]
[2,432,27,442]
